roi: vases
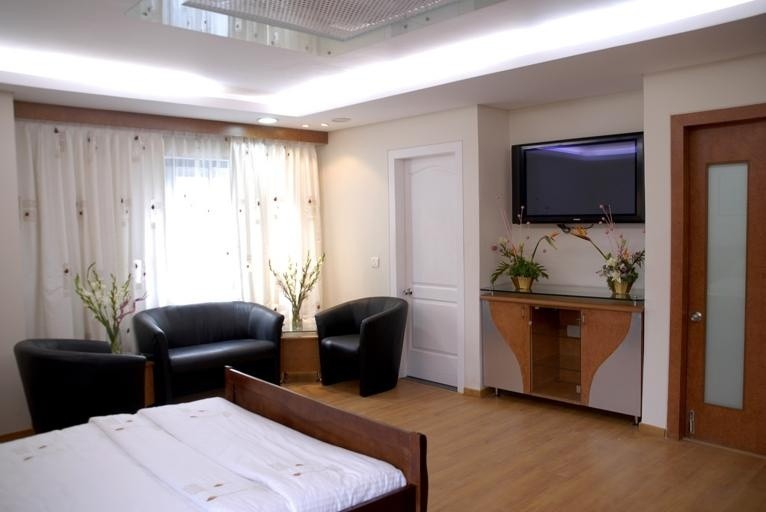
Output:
[510,276,534,294]
[607,279,635,295]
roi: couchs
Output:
[134,300,284,404]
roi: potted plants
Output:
[74,261,148,354]
[268,252,326,332]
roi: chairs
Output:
[314,296,408,397]
[14,340,147,435]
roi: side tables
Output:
[279,331,322,383]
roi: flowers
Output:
[487,193,561,285]
[561,201,646,288]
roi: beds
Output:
[1,365,429,512]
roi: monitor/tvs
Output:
[510,130,644,225]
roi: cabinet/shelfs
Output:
[481,291,643,427]
